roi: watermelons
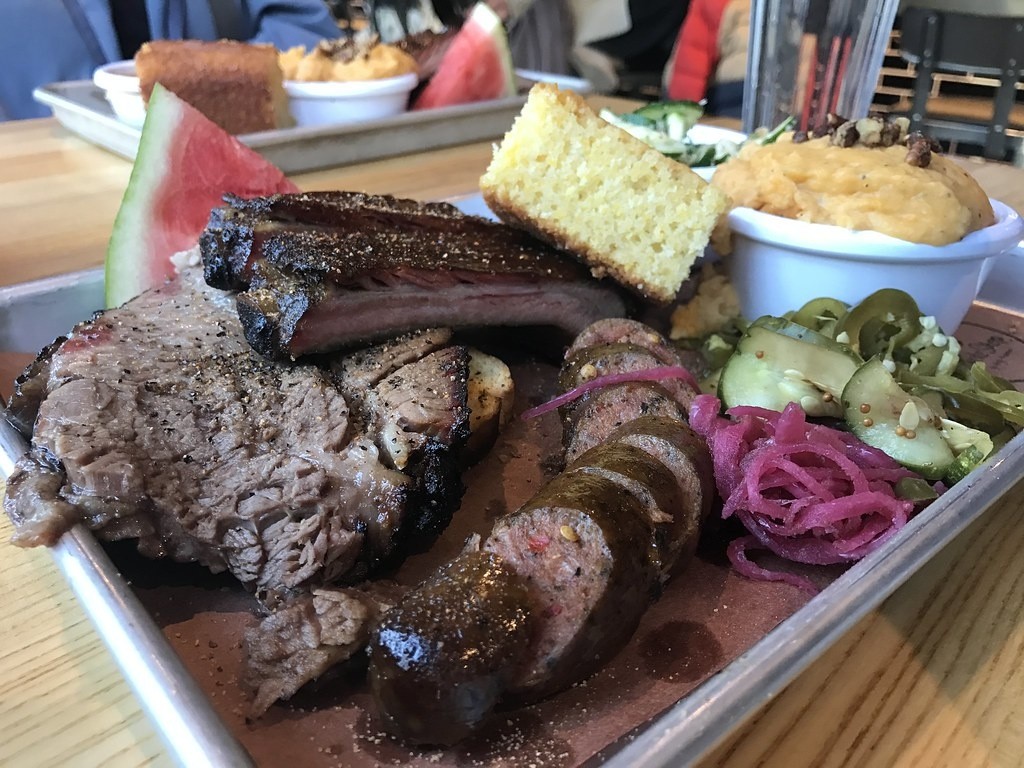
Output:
[103,81,299,305]
[412,0,517,110]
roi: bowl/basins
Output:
[281,72,418,129]
[686,125,749,268]
[728,194,1024,336]
[93,60,147,131]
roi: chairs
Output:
[896,8,1024,162]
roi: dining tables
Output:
[0,46,1024,768]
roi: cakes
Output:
[135,40,299,134]
[476,84,721,308]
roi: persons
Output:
[0,0,348,122]
[365,1,752,124]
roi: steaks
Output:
[6,187,627,727]
